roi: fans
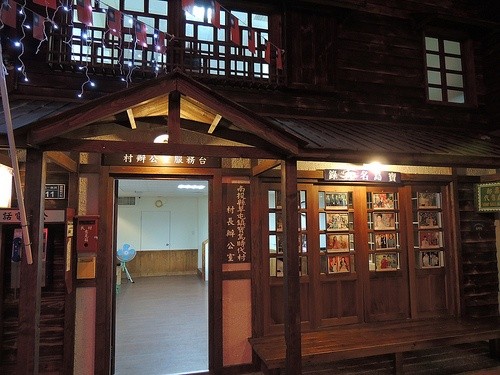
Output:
[115,242,137,286]
